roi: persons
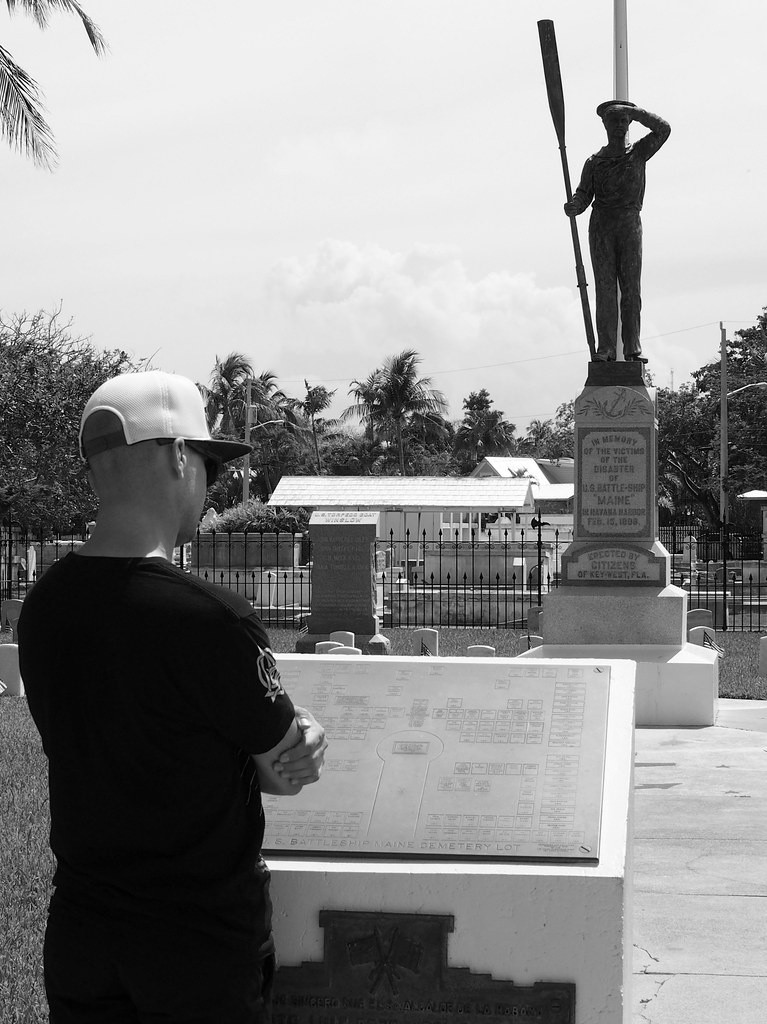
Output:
[564,100,671,363]
[17,371,328,1024]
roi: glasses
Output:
[157,438,221,488]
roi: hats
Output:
[78,372,254,464]
